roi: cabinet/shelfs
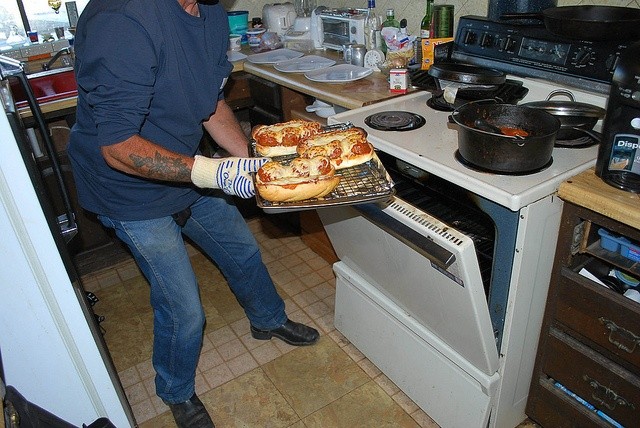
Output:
[526,169,640,428]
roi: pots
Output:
[517,88,607,143]
[452,100,562,175]
[500,6,639,37]
[427,58,530,106]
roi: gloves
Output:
[190,155,272,199]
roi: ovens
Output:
[313,6,370,50]
[313,149,537,428]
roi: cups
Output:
[345,44,365,67]
[228,34,243,51]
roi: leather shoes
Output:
[251,319,320,345]
[162,392,215,428]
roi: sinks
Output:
[8,78,52,108]
[46,69,78,100]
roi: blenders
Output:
[290,0,318,45]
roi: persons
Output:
[64,0,319,428]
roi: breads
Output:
[251,117,322,158]
[294,126,375,170]
[254,155,341,203]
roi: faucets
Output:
[42,46,73,71]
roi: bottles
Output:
[251,16,262,29]
[379,8,401,56]
[364,0,383,52]
[420,1,436,39]
[433,5,454,38]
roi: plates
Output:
[303,62,374,85]
[273,53,339,74]
[226,49,247,62]
[246,47,306,64]
